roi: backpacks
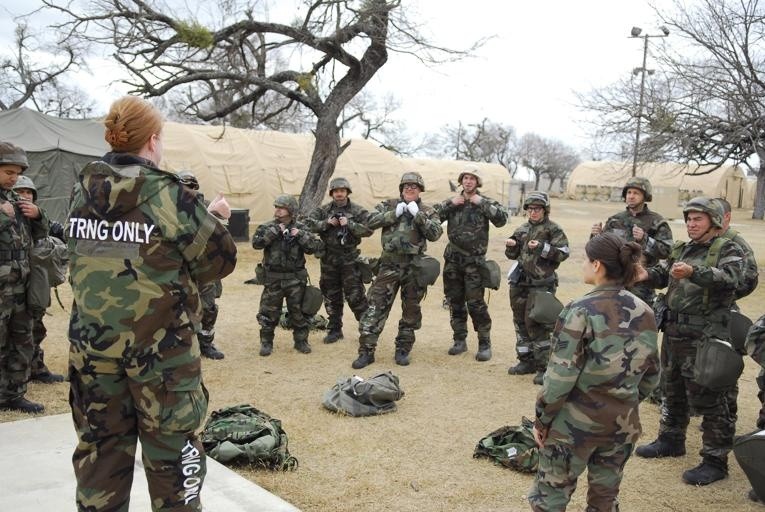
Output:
[472,414,543,475]
[196,401,299,473]
[319,370,406,417]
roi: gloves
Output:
[395,201,407,218]
[407,200,419,217]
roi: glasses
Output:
[402,183,419,189]
[527,207,544,212]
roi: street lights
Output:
[624,25,672,178]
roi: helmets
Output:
[458,168,482,187]
[523,191,551,213]
[620,176,652,202]
[398,171,425,192]
[176,170,199,190]
[328,177,352,196]
[682,196,724,230]
[12,175,38,202]
[273,195,299,218]
[0,142,29,171]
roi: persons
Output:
[249,191,314,358]
[174,173,226,359]
[10,174,66,386]
[0,140,47,413]
[503,191,570,390]
[306,176,373,343]
[708,198,759,451]
[350,172,442,369]
[527,231,661,511]
[740,313,765,506]
[588,176,674,306]
[62,96,237,512]
[431,165,508,362]
[626,196,745,485]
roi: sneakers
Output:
[198,339,225,360]
[447,338,468,355]
[636,432,687,459]
[0,397,44,414]
[507,363,536,375]
[294,342,311,354]
[533,372,544,385]
[30,371,64,384]
[259,339,273,356]
[394,349,409,366]
[351,351,375,369]
[322,327,343,343]
[476,344,491,362]
[679,460,729,486]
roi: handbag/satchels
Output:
[27,243,52,314]
[732,429,765,502]
[301,284,324,315]
[407,253,441,291]
[694,307,746,394]
[731,310,753,357]
[28,234,68,285]
[527,294,564,328]
[477,260,501,290]
[356,254,373,284]
[49,218,67,244]
